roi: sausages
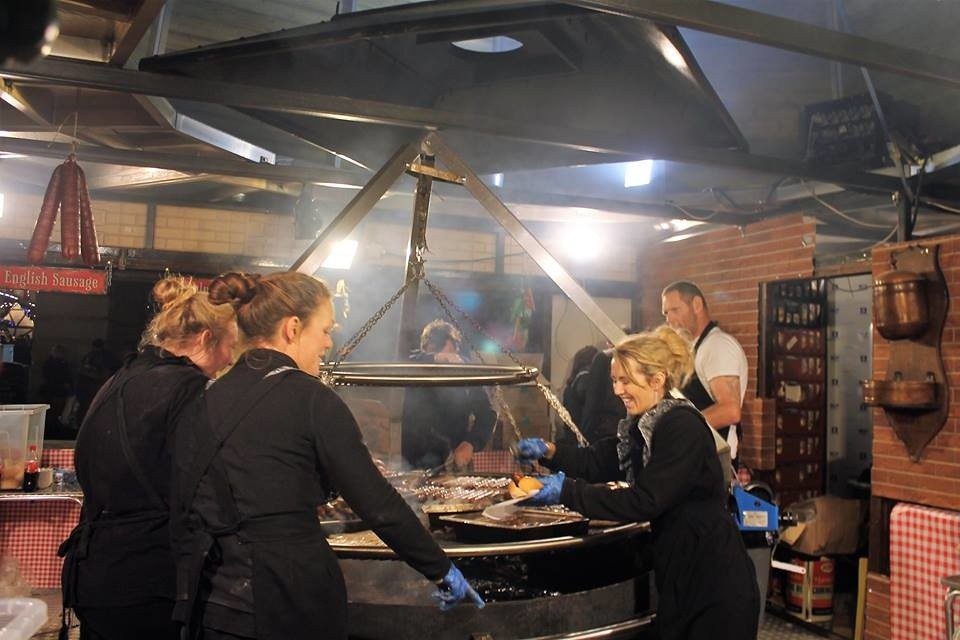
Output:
[317,495,354,521]
[27,152,101,267]
[413,471,513,504]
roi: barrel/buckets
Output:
[786,552,834,613]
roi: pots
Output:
[861,371,935,407]
[872,259,929,338]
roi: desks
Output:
[0,491,84,505]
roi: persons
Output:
[580,324,640,447]
[166,270,487,640]
[511,325,760,640]
[399,317,497,475]
[561,343,599,434]
[55,274,238,639]
[660,279,750,479]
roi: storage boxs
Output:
[0,596,48,640]
[1,404,51,491]
[783,495,861,555]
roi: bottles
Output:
[24,445,40,493]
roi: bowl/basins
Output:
[39,468,53,488]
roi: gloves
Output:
[514,470,565,507]
[518,438,547,459]
[433,561,485,614]
